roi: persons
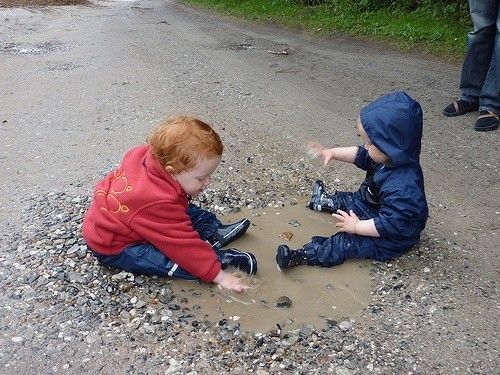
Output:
[276,90,428,270]
[441,0,500,132]
[81,117,258,293]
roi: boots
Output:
[276,244,307,272]
[309,180,338,212]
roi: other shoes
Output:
[220,248,257,276]
[207,218,250,250]
[474,110,499,131]
[443,99,479,116]
